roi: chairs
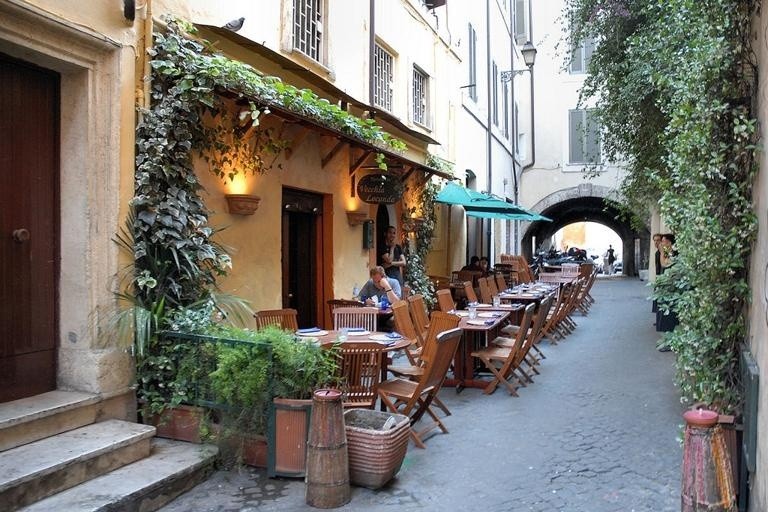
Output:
[374,327,465,448]
[326,254,599,398]
[332,340,382,416]
[254,309,298,334]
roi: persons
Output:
[480,257,496,276]
[377,226,406,286]
[357,265,402,332]
[652,233,663,326]
[605,245,616,275]
[458,255,487,288]
[656,234,679,332]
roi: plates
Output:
[302,336,320,344]
[368,334,403,340]
[296,330,328,336]
[348,330,370,336]
[473,303,512,308]
[505,285,550,297]
[466,320,485,326]
[477,313,496,317]
[456,312,468,317]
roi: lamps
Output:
[501,38,537,83]
[345,209,366,226]
[226,192,261,215]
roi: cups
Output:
[469,307,477,319]
[535,280,543,288]
[371,297,378,305]
[337,328,347,341]
[493,296,500,306]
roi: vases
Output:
[342,408,412,491]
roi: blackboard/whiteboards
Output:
[357,174,404,204]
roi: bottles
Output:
[381,295,387,310]
[352,282,359,301]
[360,294,366,304]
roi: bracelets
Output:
[386,288,392,292]
